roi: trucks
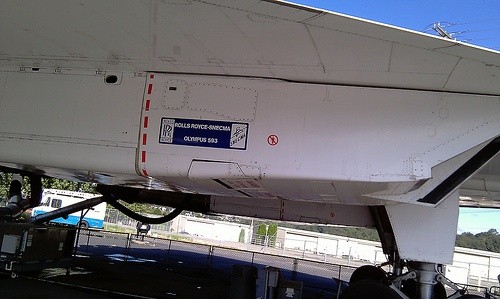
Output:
[29,188,107,230]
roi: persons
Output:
[0,180,24,207]
